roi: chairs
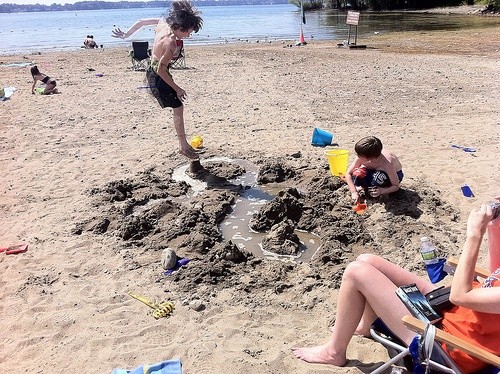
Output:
[128,41,152,71]
[356,256,500,374]
[168,40,186,69]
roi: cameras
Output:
[488,203,500,221]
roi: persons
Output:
[291,196,500,374]
[84,35,98,48]
[31,65,61,95]
[344,136,404,205]
[111,0,203,159]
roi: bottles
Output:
[420,237,439,266]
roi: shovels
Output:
[353,200,367,215]
[0,243,28,254]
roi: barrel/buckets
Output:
[311,128,333,147]
[326,149,349,177]
[191,135,203,149]
